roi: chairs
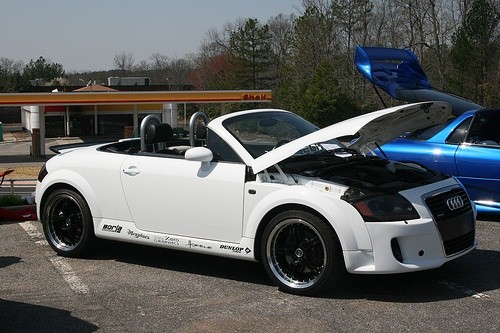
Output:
[140,114,179,155]
[189,111,209,147]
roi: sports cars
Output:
[351,46,499,218]
[34,99,478,296]
